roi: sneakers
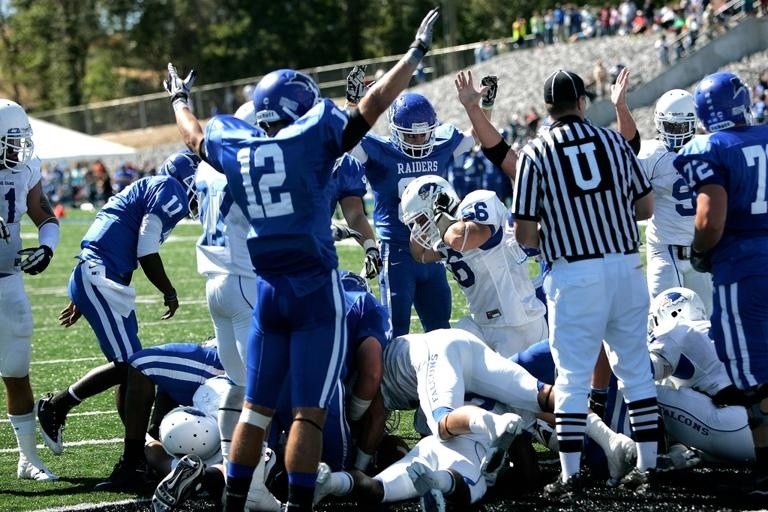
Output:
[244,484,288,512]
[406,460,449,512]
[479,412,522,479]
[152,454,206,512]
[35,395,65,455]
[313,462,332,505]
[545,432,701,498]
[17,459,59,480]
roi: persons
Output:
[0,95,64,482]
[37,1,767,512]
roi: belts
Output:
[566,248,639,263]
[678,246,695,261]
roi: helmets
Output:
[234,69,322,136]
[653,73,751,151]
[0,99,34,173]
[161,150,202,221]
[401,175,460,250]
[388,93,438,161]
[648,287,707,342]
[159,406,221,460]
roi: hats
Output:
[544,70,598,104]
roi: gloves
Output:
[690,243,712,273]
[332,223,363,241]
[345,63,377,104]
[17,245,54,275]
[409,6,441,56]
[364,247,383,279]
[163,62,198,105]
[0,217,12,244]
[432,191,455,216]
[481,76,498,108]
